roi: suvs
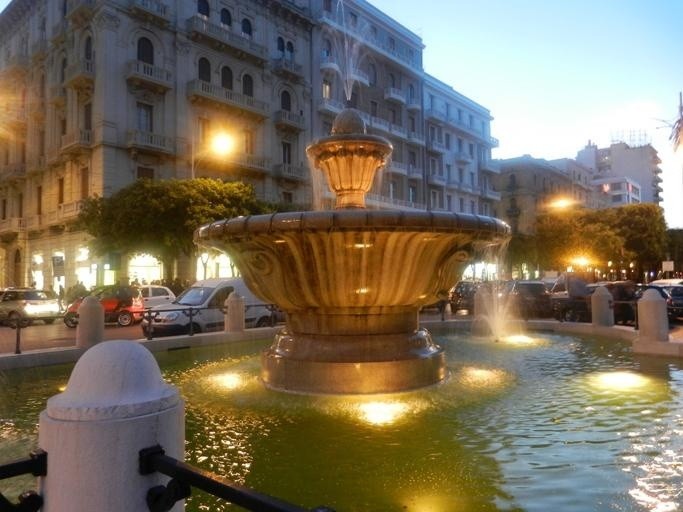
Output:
[141,278,274,337]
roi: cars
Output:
[0,288,63,326]
[64,286,145,328]
[449,273,683,328]
[139,284,177,308]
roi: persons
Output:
[31,281,87,312]
[115,278,192,298]
